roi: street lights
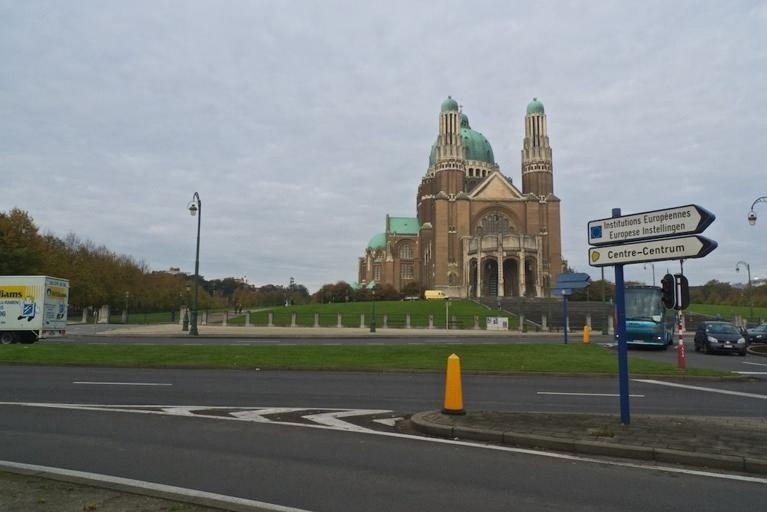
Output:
[747,195,766,226]
[181,283,191,331]
[369,290,376,332]
[185,189,203,337]
[643,262,655,286]
[735,260,752,321]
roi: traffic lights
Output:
[659,272,676,310]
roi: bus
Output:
[615,282,675,352]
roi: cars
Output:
[399,294,420,301]
[693,320,766,357]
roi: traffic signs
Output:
[587,201,717,247]
[587,233,720,268]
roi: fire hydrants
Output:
[581,324,592,344]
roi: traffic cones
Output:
[439,351,469,416]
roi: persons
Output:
[239,304,242,314]
[234,303,238,315]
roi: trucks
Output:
[0,274,72,345]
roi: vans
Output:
[423,289,449,301]
